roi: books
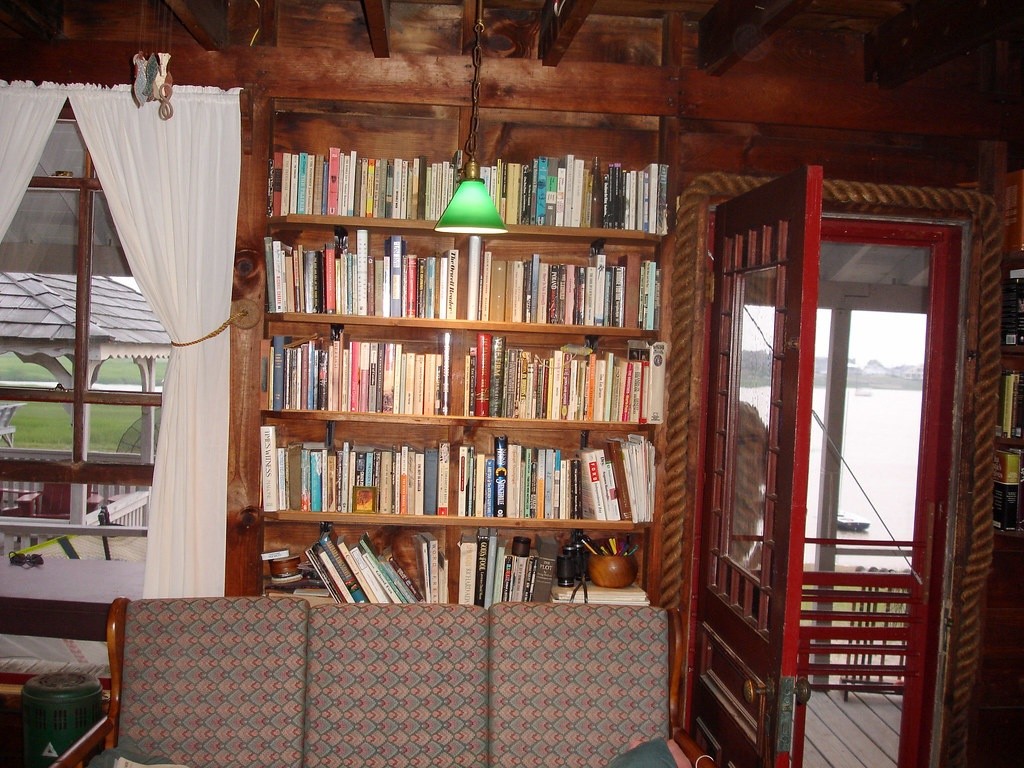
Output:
[264,148,670,522]
[991,169,1024,530]
[532,535,561,602]
[305,525,539,603]
[549,577,651,606]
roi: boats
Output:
[837,513,870,531]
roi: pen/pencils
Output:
[580,533,639,556]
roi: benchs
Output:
[52,596,716,768]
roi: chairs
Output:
[14,483,103,519]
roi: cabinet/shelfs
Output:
[254,98,682,603]
[991,251,1024,553]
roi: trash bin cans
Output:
[20,669,103,768]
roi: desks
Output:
[0,555,147,641]
[108,494,128,503]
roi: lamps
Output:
[433,0,508,234]
